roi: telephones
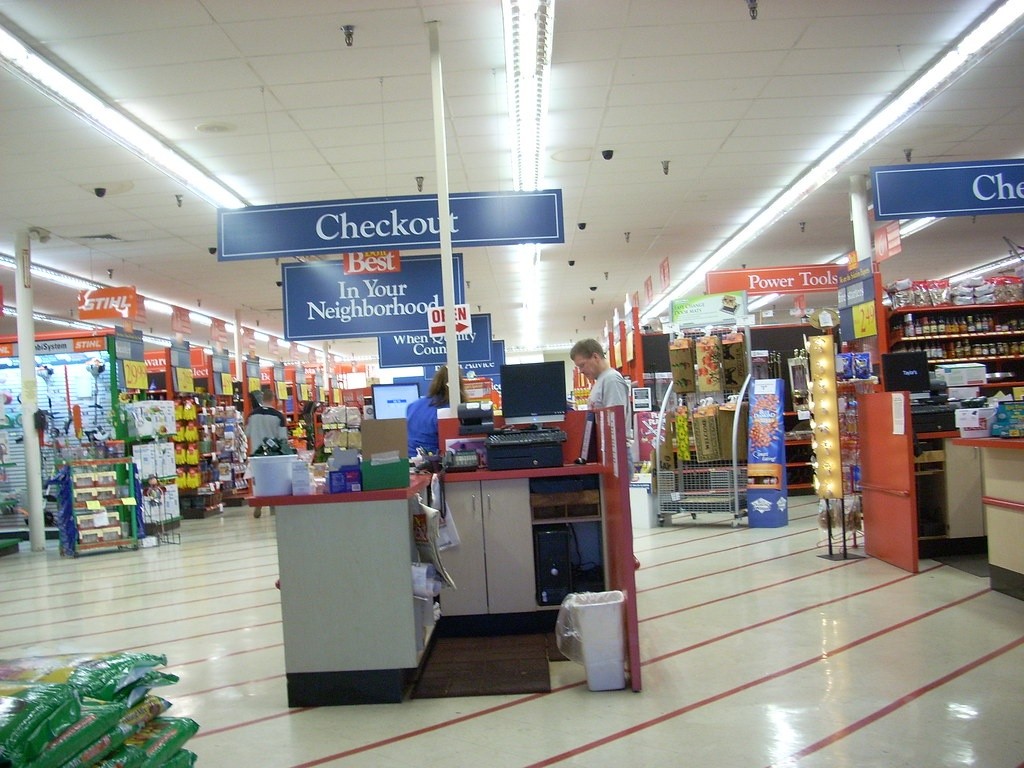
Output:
[442,449,479,472]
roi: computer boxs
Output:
[535,525,573,606]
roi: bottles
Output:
[890,313,1024,337]
[72,440,124,458]
[891,338,971,358]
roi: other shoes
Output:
[254,504,262,519]
[633,555,640,569]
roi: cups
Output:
[217,342,223,353]
[125,318,133,333]
[249,351,254,359]
[178,332,185,344]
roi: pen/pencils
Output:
[416,445,428,455]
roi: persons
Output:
[404,365,467,466]
[569,338,641,571]
[244,389,288,517]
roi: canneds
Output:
[971,340,1024,357]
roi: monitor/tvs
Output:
[372,383,420,419]
[500,361,567,428]
[881,351,932,404]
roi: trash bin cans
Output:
[573,590,627,691]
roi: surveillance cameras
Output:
[207,248,217,254]
[578,223,586,230]
[589,287,597,291]
[94,188,106,197]
[276,281,282,287]
[601,150,614,160]
[568,260,575,266]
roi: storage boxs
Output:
[934,361,988,387]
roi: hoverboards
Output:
[37,365,64,438]
[25,463,56,527]
[82,357,111,442]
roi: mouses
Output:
[574,458,587,464]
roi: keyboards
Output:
[483,431,567,447]
[912,405,958,414]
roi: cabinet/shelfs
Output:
[273,470,610,704]
[888,301,1024,388]
[740,323,833,495]
[131,371,333,521]
